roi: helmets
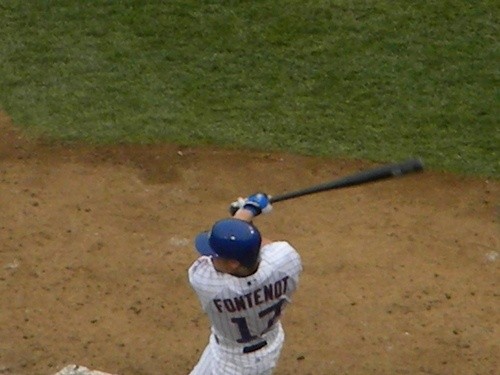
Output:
[195,218,261,267]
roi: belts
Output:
[242,342,268,353]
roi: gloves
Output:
[243,192,270,216]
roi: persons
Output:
[187,191,303,375]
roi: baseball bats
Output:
[229,159,423,214]
